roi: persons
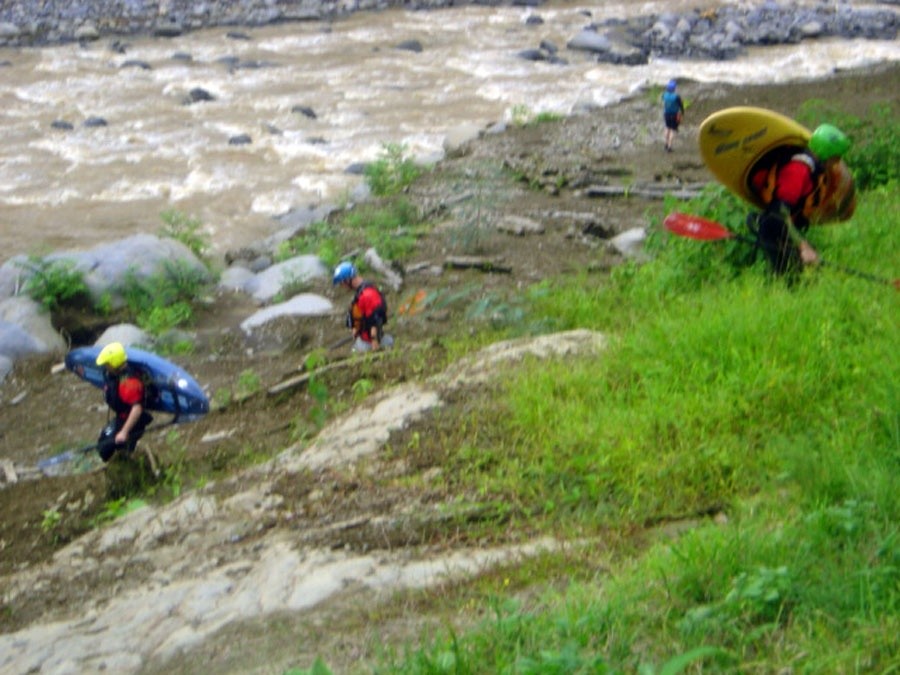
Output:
[761,123,849,291]
[95,343,154,463]
[333,263,387,353]
[662,80,685,152]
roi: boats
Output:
[64,345,210,415]
[695,105,857,223]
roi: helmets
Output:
[666,81,676,92]
[96,343,127,370]
[808,122,853,160]
[333,261,356,285]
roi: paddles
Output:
[331,288,437,351]
[664,208,900,292]
[37,411,198,467]
